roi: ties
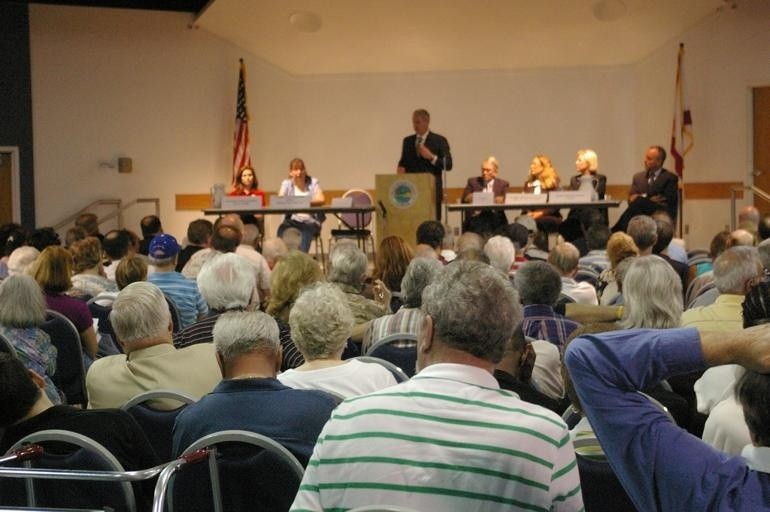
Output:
[416,136,421,156]
[647,173,655,189]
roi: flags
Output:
[671,45,695,179]
[231,66,251,189]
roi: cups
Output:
[455,197,461,205]
[604,194,611,201]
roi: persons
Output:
[611,147,678,233]
[276,158,326,254]
[462,156,510,233]
[397,109,452,222]
[2,207,770,512]
[521,155,562,220]
[228,166,265,225]
[568,150,608,225]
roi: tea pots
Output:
[576,175,599,201]
[209,183,226,208]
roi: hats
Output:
[149,233,181,259]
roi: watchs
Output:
[430,154,434,161]
